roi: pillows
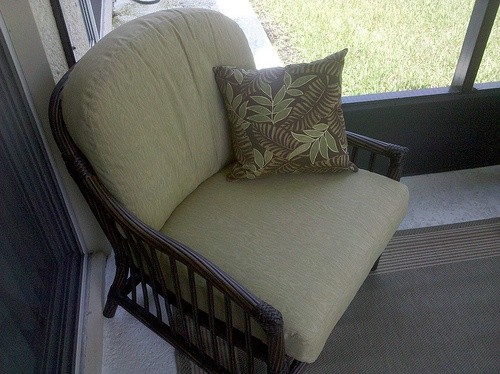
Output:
[212,47,359,182]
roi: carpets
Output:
[171,216,499,374]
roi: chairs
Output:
[49,7,410,374]
